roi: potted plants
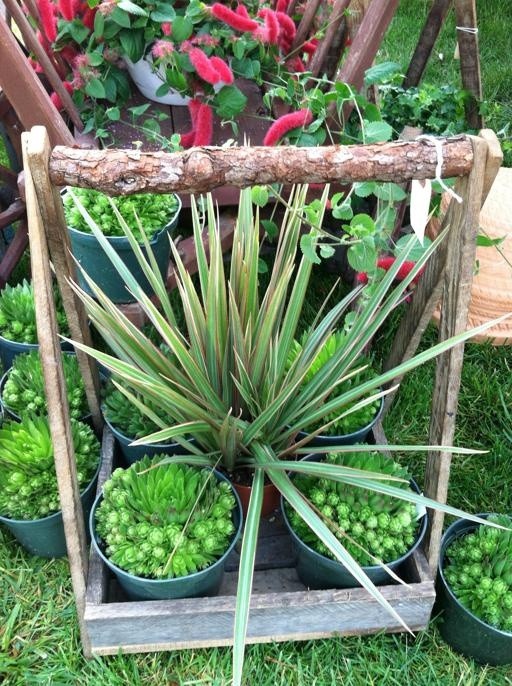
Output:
[99,381,198,463]
[57,186,183,308]
[0,410,101,563]
[47,188,509,686]
[1,354,95,432]
[284,447,429,591]
[285,348,387,447]
[88,460,243,597]
[429,510,512,667]
[1,275,71,367]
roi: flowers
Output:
[24,0,353,153]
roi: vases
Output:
[122,44,231,106]
